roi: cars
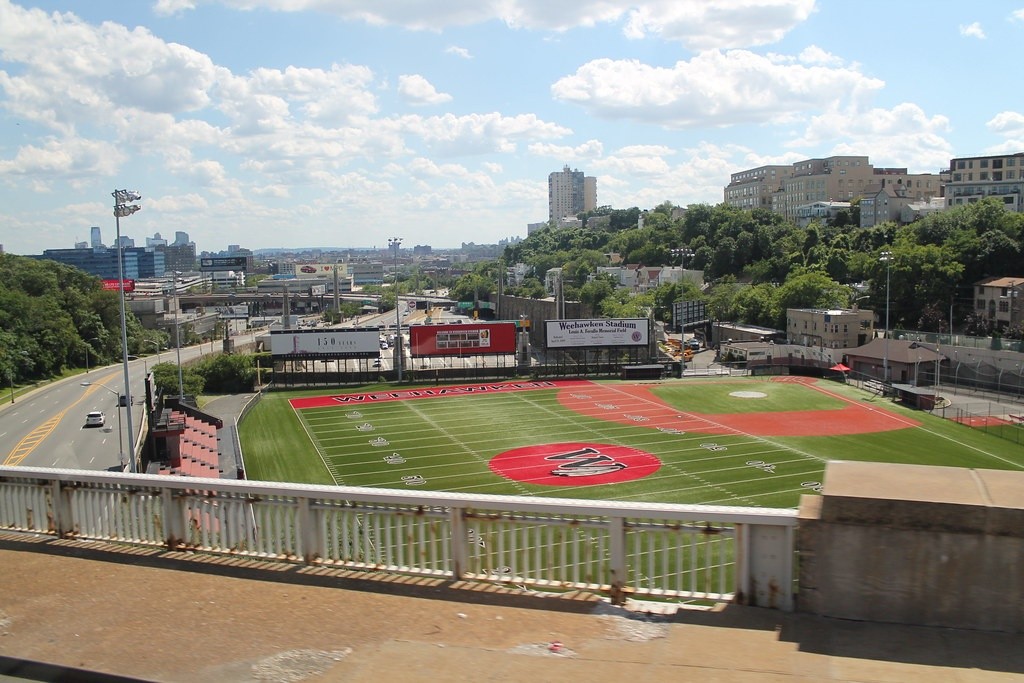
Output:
[374,359,381,367]
[120,395,134,407]
[381,343,388,349]
[301,265,318,273]
[405,308,409,312]
[299,320,323,326]
[688,339,700,351]
[85,411,106,427]
[403,313,408,316]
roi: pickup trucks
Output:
[663,338,694,362]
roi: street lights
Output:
[85,338,98,373]
[142,339,161,366]
[80,382,124,473]
[172,270,183,399]
[879,251,896,382]
[388,237,404,383]
[670,248,696,369]
[9,351,28,404]
[110,189,143,492]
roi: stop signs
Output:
[407,301,417,311]
